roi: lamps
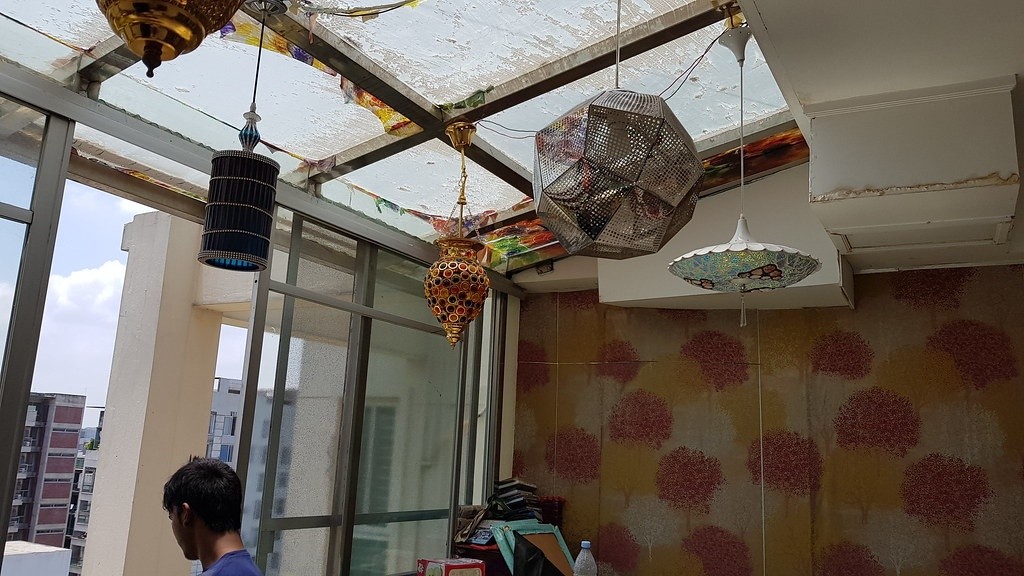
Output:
[532,0,704,261]
[197,0,288,272]
[423,121,490,350]
[95,0,247,78]
[667,27,822,326]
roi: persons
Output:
[163,454,264,576]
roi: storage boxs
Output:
[417,558,486,576]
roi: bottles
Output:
[572,540,598,576]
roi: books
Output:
[492,477,542,520]
